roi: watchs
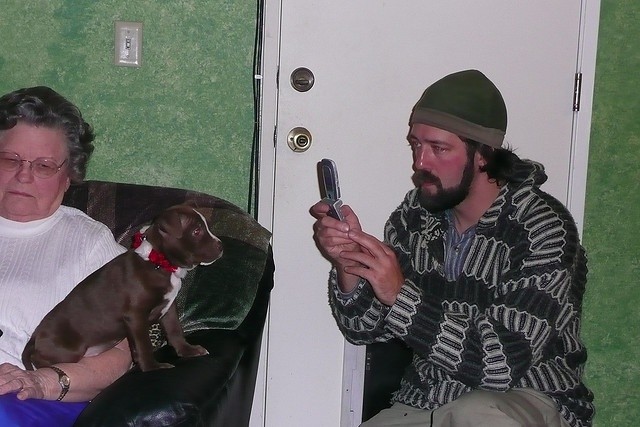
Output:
[45,366,71,402]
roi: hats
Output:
[408,69,508,150]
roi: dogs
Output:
[22,199,225,374]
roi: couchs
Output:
[60,179,276,427]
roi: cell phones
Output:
[317,158,344,222]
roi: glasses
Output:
[0,150,68,179]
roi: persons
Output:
[308,68,598,426]
[1,84,140,426]
